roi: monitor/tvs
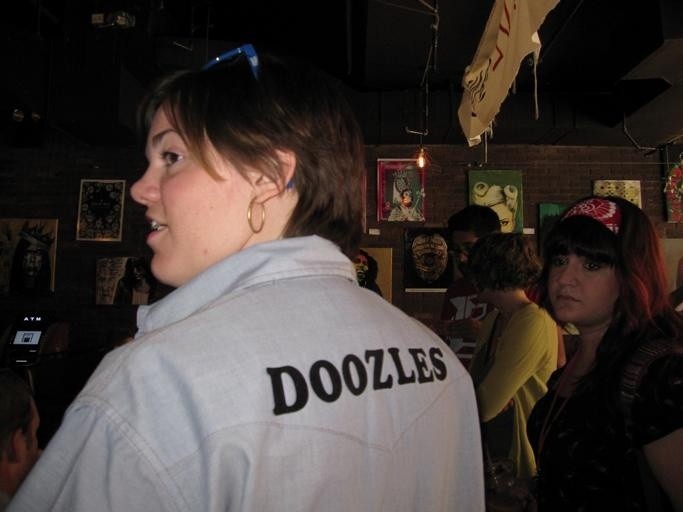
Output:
[12,330,42,345]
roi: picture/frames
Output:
[76,178,126,243]
[375,157,429,223]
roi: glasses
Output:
[193,43,295,189]
[446,244,472,256]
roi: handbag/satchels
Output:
[486,461,537,511]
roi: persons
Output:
[2,50,486,512]
[8,231,53,296]
[112,257,158,306]
[0,366,40,512]
[350,246,385,296]
[387,189,421,222]
